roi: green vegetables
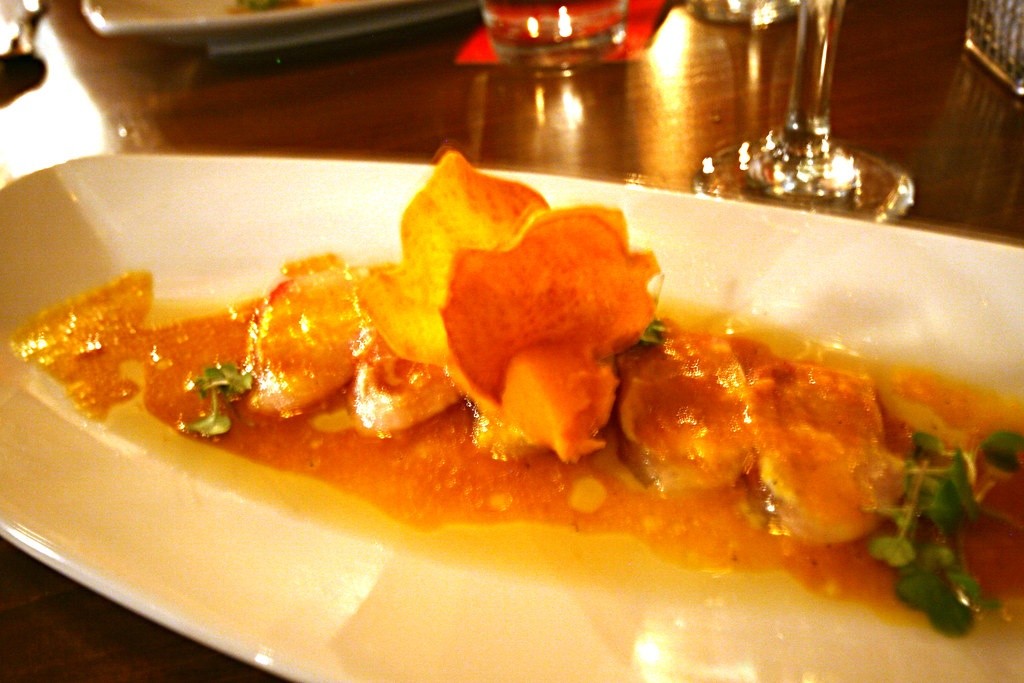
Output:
[639,319,667,348]
[189,365,256,439]
[859,429,1024,635]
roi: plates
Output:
[1,154,1023,682]
[81,1,479,56]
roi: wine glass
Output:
[690,0,913,223]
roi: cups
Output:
[479,0,627,67]
[963,0,1023,96]
